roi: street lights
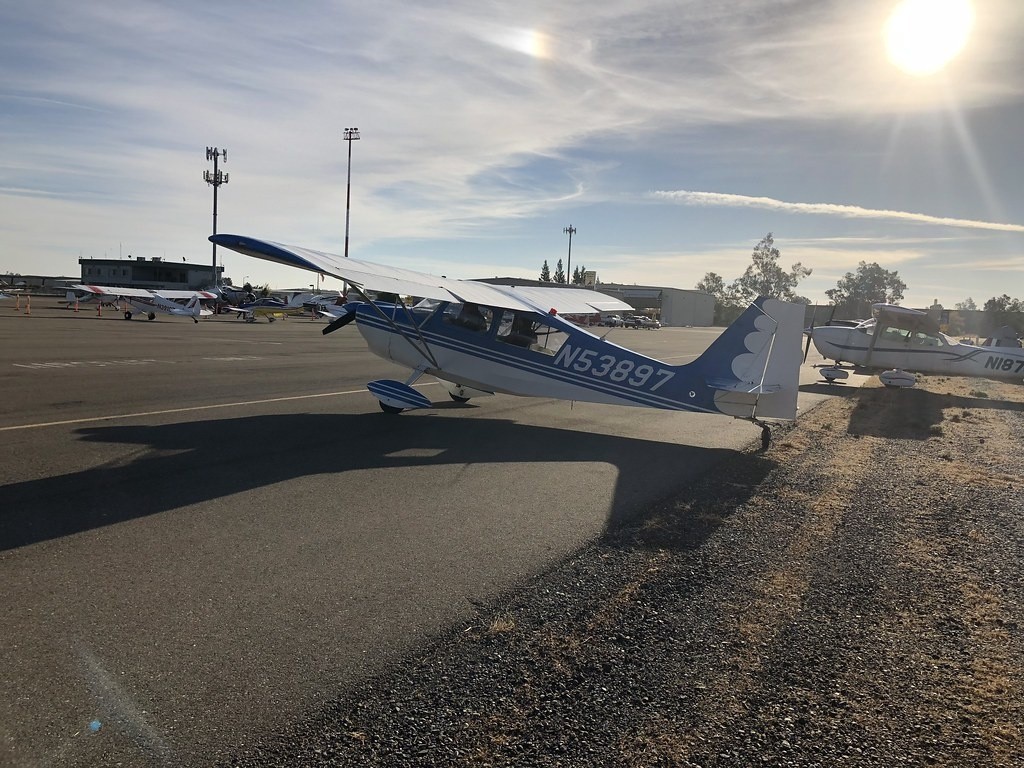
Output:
[562,224,577,284]
[202,145,230,287]
[340,125,362,297]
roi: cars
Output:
[603,313,663,332]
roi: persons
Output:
[236,299,247,319]
[633,320,641,330]
[463,307,487,332]
[313,303,330,320]
[498,316,538,350]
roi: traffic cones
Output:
[73,297,79,312]
[96,301,102,316]
[24,295,31,314]
[15,294,20,311]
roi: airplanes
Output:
[56,282,355,323]
[205,231,803,455]
[802,302,1024,390]
